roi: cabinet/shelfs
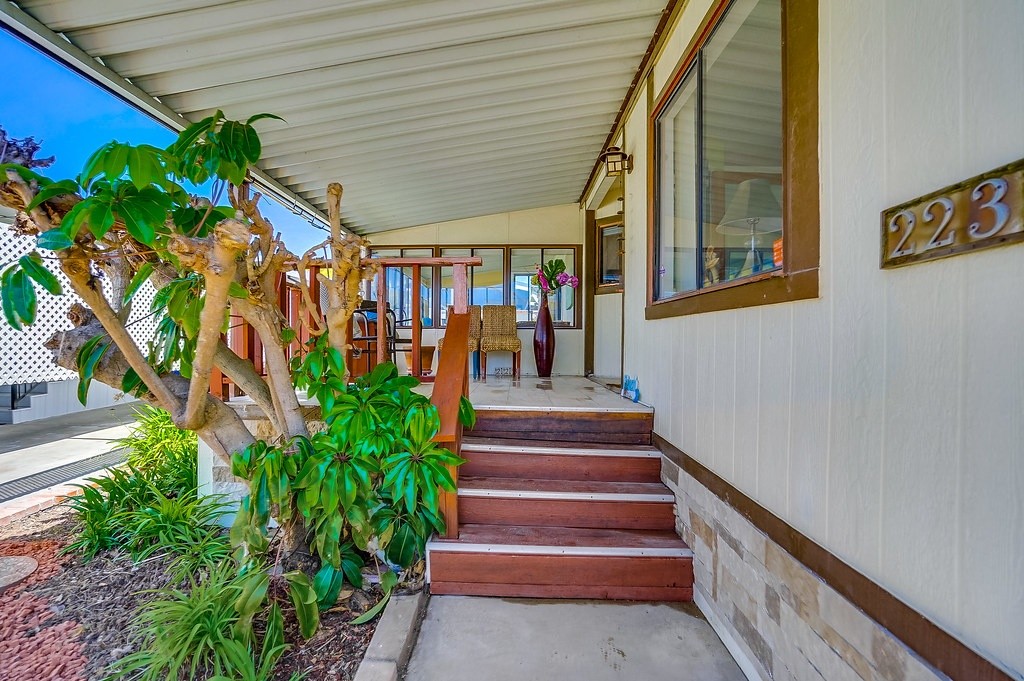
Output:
[349,308,397,385]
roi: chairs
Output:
[480,305,521,382]
[436,304,481,379]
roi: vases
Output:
[403,345,435,374]
[534,296,555,377]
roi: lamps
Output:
[716,178,782,277]
[598,144,635,177]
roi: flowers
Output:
[529,258,578,297]
